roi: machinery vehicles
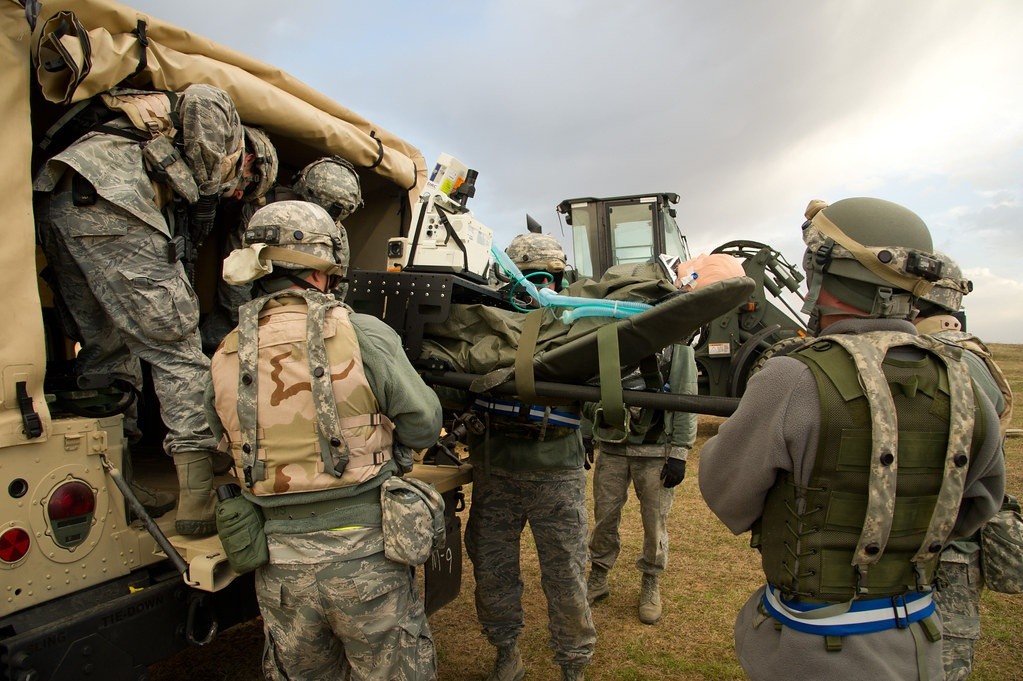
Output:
[525,193,810,398]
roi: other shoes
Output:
[172,451,224,535]
[122,437,177,519]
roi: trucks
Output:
[0,1,495,681]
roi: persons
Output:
[904,251,1013,681]
[585,338,699,624]
[204,200,442,681]
[463,233,597,681]
[427,254,747,373]
[32,84,363,539]
[700,201,1004,681]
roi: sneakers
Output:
[586,561,610,607]
[638,573,662,624]
[559,667,586,680]
[485,641,526,681]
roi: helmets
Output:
[911,252,973,314]
[289,153,365,223]
[502,231,567,273]
[241,125,279,206]
[238,199,351,278]
[801,196,943,292]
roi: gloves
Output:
[660,456,687,489]
[582,438,595,470]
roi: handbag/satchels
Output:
[977,508,1023,594]
[379,476,447,566]
